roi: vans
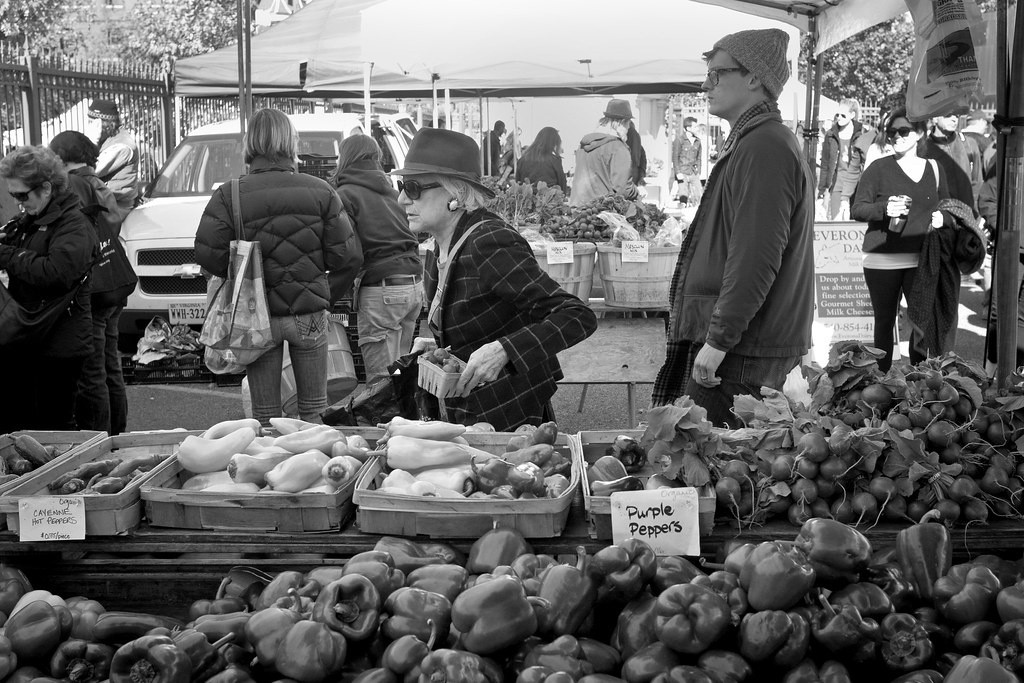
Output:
[120,112,418,355]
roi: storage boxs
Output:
[272,316,358,416]
[0,429,111,525]
[575,429,717,542]
[0,428,207,537]
[417,348,469,398]
[139,424,392,534]
[217,369,247,387]
[136,355,215,384]
[528,241,596,306]
[121,351,138,385]
[597,241,684,310]
[352,430,578,537]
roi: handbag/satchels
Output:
[0,255,104,377]
[198,176,276,376]
[72,171,139,310]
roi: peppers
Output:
[0,341,1024,683]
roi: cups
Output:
[889,194,913,232]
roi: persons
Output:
[47,131,131,433]
[1,145,111,434]
[383,127,599,430]
[418,93,1023,374]
[85,99,138,233]
[648,26,819,428]
[192,108,356,428]
[330,132,423,389]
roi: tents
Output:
[172,2,802,181]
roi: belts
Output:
[360,274,423,287]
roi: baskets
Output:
[352,427,582,539]
[1,430,206,537]
[139,426,387,535]
[416,352,467,399]
[121,356,215,385]
[577,430,717,540]
[0,430,108,526]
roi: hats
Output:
[603,99,635,119]
[713,27,791,101]
[385,126,496,200]
[966,109,990,125]
[87,98,119,122]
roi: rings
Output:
[701,376,708,380]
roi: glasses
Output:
[704,68,748,86]
[885,127,915,138]
[8,184,42,201]
[835,113,847,118]
[397,180,442,200]
